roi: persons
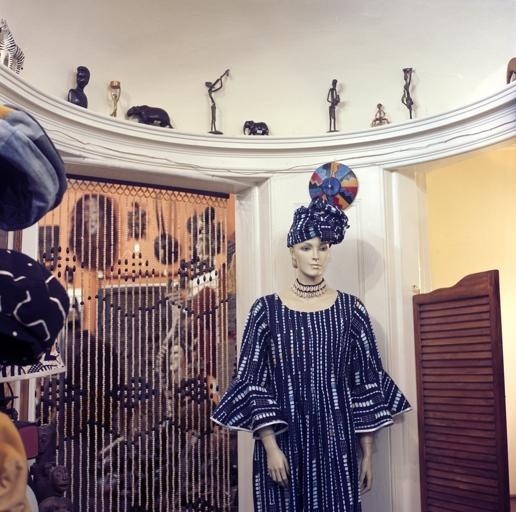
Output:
[209,194,413,512]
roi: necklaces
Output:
[289,277,328,299]
